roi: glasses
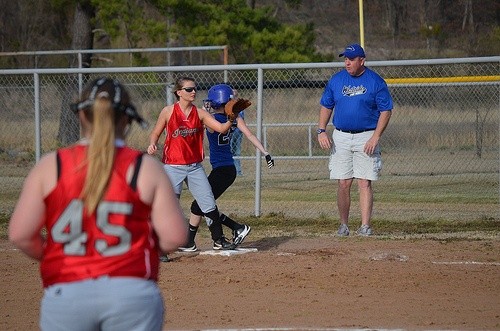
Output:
[177,87,197,92]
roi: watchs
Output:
[317,129,325,134]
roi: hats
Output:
[78,77,133,109]
[338,44,366,59]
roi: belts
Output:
[335,128,376,133]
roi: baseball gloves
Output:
[225,98,251,120]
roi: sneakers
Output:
[213,238,237,251]
[230,224,251,245]
[160,254,169,262]
[353,225,372,236]
[336,224,350,237]
[176,242,197,252]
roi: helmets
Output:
[206,85,235,106]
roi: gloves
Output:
[264,155,274,169]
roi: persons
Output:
[177,84,273,252]
[316,44,394,237]
[9,76,189,331]
[146,76,251,252]
